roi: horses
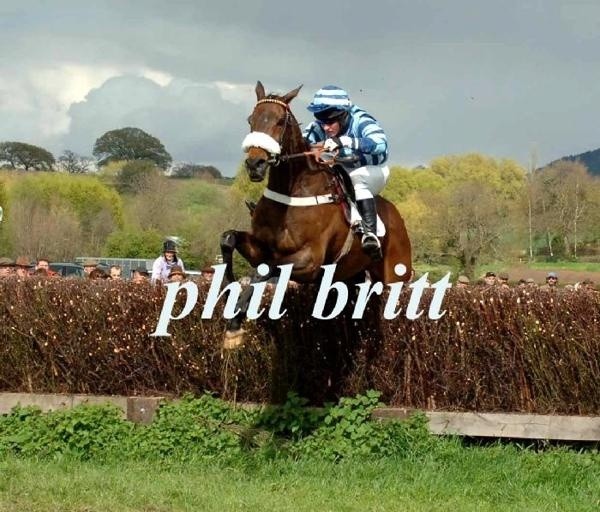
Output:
[219,81,412,349]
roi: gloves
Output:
[323,135,353,152]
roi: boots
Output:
[355,196,384,265]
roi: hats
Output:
[14,256,35,268]
[162,240,177,254]
[0,257,17,267]
[82,260,98,268]
[200,266,216,273]
[167,266,187,280]
[546,272,558,280]
[486,272,497,279]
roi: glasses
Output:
[316,115,337,125]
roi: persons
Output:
[300,87,391,266]
[0,242,220,283]
[455,272,596,293]
[406,269,416,285]
